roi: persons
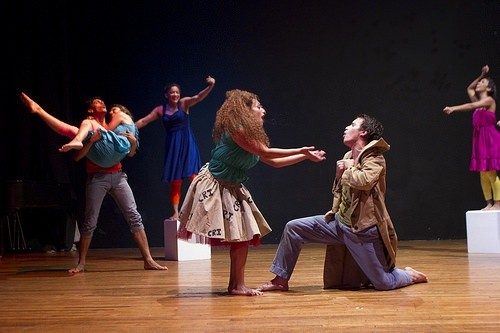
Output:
[254,113,428,292]
[443,64,500,211]
[176,89,326,296]
[134,76,216,221]
[68,96,168,273]
[20,92,140,169]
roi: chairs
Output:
[0,179,65,255]
